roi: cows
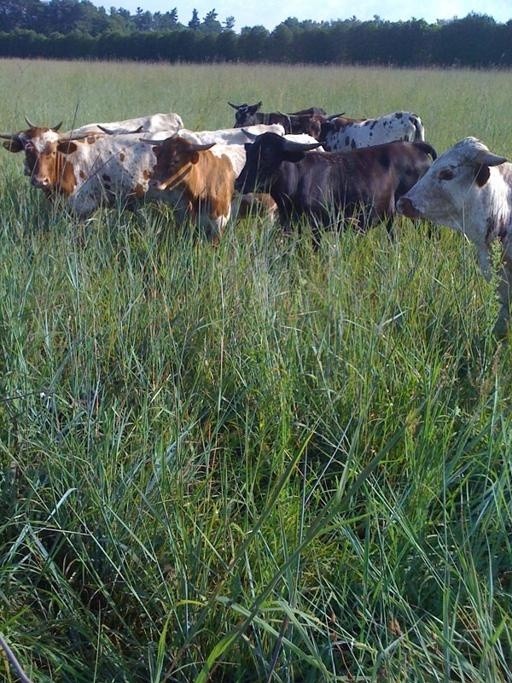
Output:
[0,116,176,221]
[65,112,185,134]
[234,129,438,256]
[137,134,325,246]
[227,102,424,152]
[395,135,512,355]
